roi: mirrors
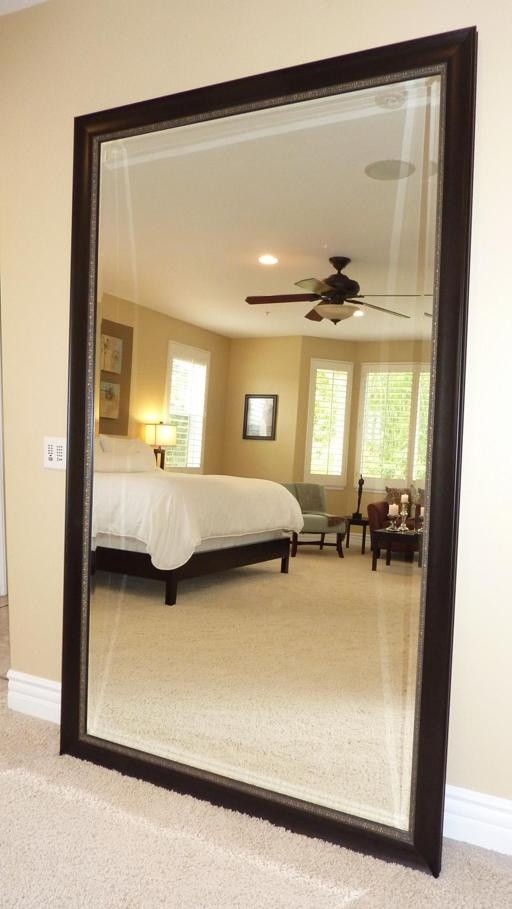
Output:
[61,27,482,882]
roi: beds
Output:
[90,435,306,606]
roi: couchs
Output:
[367,501,427,551]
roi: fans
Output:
[243,256,422,322]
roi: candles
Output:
[401,495,408,503]
[389,505,399,514]
[421,507,425,515]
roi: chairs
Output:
[283,482,347,559]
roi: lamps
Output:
[143,421,177,473]
[314,256,359,323]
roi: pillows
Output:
[385,486,411,507]
[92,434,114,473]
[100,435,156,472]
[418,488,425,504]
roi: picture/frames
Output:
[242,393,277,440]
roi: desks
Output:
[346,517,372,554]
[372,528,424,571]
